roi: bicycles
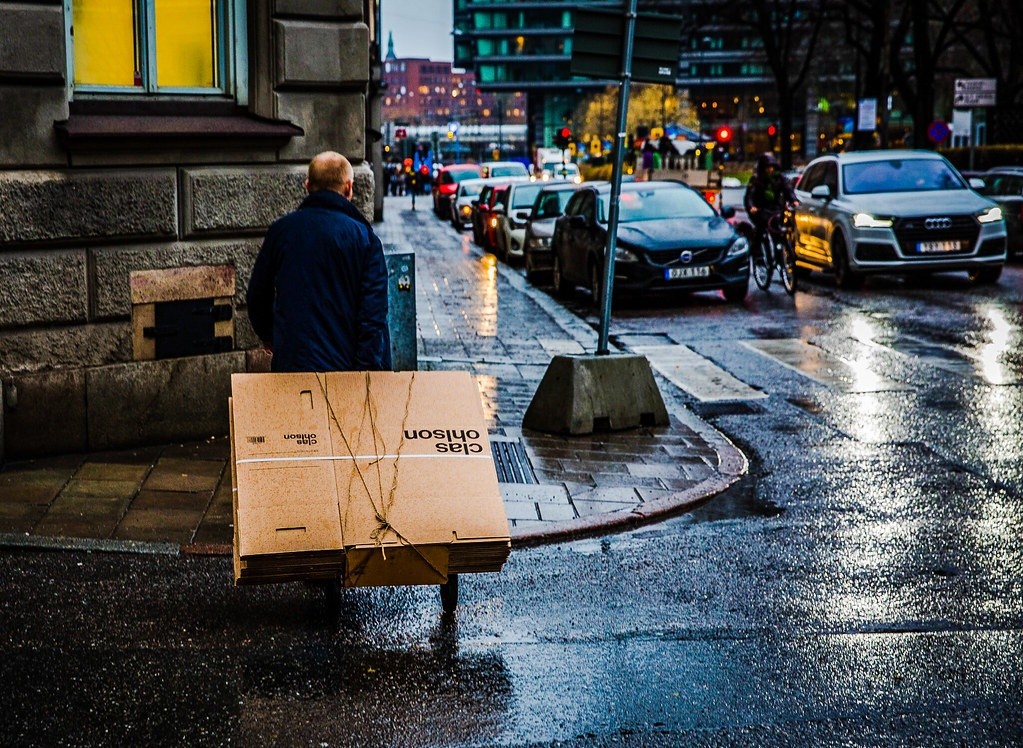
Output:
[748,205,803,294]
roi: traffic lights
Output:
[767,126,776,137]
[719,127,729,157]
[556,127,570,146]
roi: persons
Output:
[627,149,638,173]
[380,163,436,200]
[246,151,390,370]
[743,154,802,267]
[820,115,929,157]
[642,143,655,183]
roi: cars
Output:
[516,179,609,284]
[791,147,1007,289]
[552,177,751,309]
[492,178,578,264]
[433,159,528,253]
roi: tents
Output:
[657,122,712,142]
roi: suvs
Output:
[973,166,1023,262]
